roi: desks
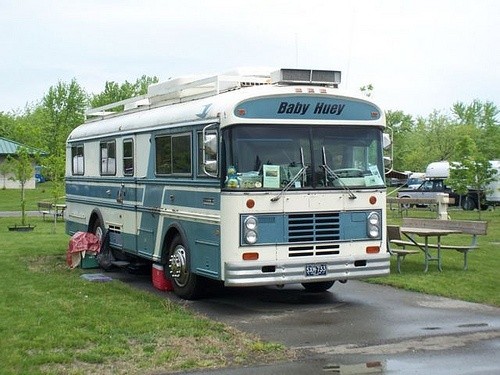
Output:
[400,226,462,272]
[53,203,67,214]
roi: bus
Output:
[408,172,426,188]
[65,67,394,300]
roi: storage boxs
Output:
[152,264,174,291]
[81,255,100,269]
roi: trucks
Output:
[397,161,500,210]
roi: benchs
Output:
[37,202,63,221]
[387,225,420,273]
[404,218,488,270]
